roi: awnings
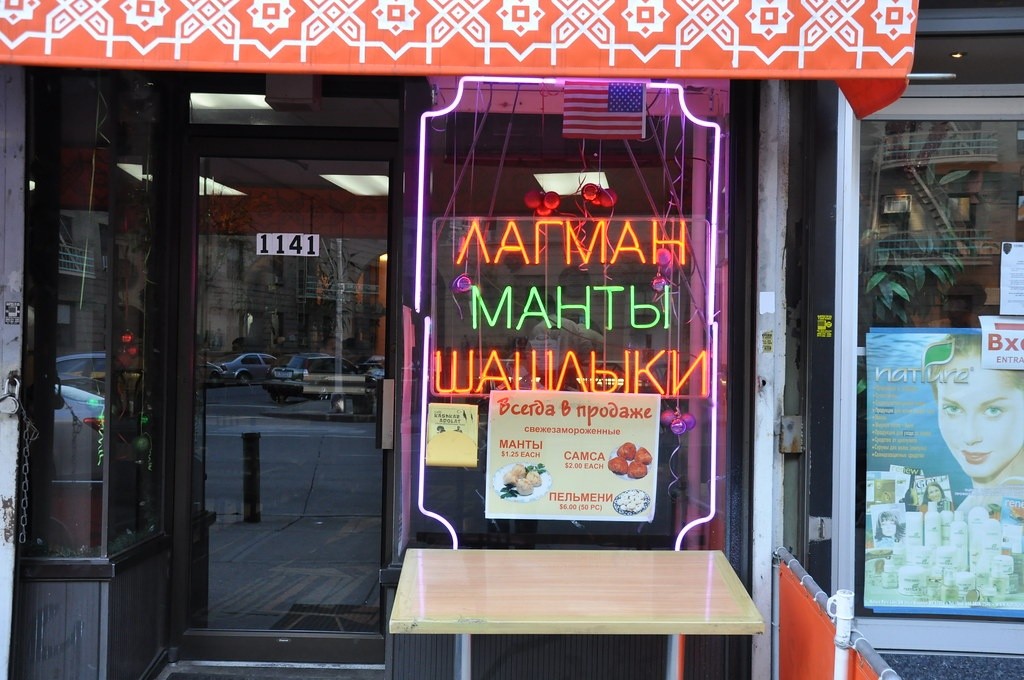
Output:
[0,1,916,120]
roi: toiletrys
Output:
[879,501,1023,605]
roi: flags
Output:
[563,82,646,138]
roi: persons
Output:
[524,291,607,389]
[877,513,904,545]
[930,333,1024,516]
[925,484,947,504]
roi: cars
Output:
[354,355,385,375]
[196,357,223,387]
[47,386,109,494]
[221,353,278,386]
[365,367,385,391]
[264,353,373,405]
[58,372,110,400]
[57,353,116,375]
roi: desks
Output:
[388,548,764,680]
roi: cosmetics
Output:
[882,501,1019,601]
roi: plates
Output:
[613,489,650,516]
[492,462,552,503]
[610,446,651,480]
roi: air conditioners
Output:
[881,194,912,212]
